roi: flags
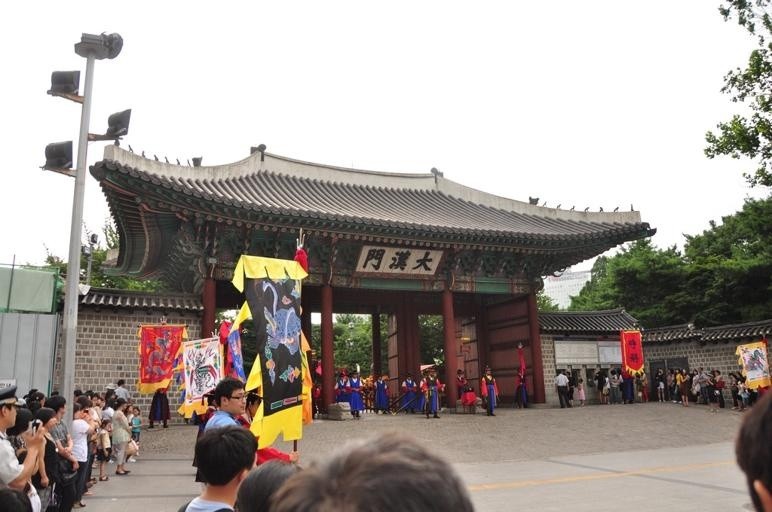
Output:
[135,255,314,451]
[517,344,528,377]
[620,328,645,377]
[735,340,770,390]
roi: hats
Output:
[0,383,26,408]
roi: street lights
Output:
[40,31,132,431]
[81,234,101,286]
[348,321,355,338]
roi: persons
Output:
[733,392,772,512]
[455,369,472,399]
[334,366,444,419]
[555,364,648,408]
[268,426,473,511]
[176,424,259,511]
[1,377,174,511]
[515,374,529,408]
[234,457,305,511]
[481,366,499,418]
[656,366,761,414]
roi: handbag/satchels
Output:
[59,461,79,485]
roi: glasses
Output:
[231,393,249,401]
